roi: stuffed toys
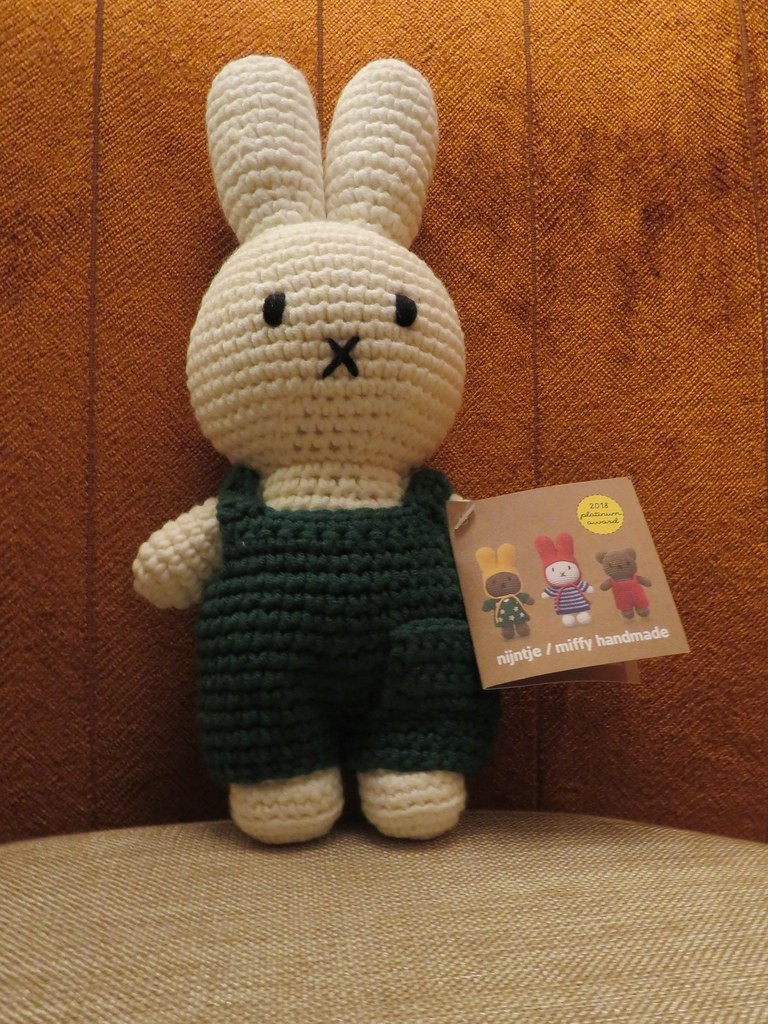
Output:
[535,531,595,627]
[596,547,654,620]
[128,46,482,847]
[475,543,537,643]
